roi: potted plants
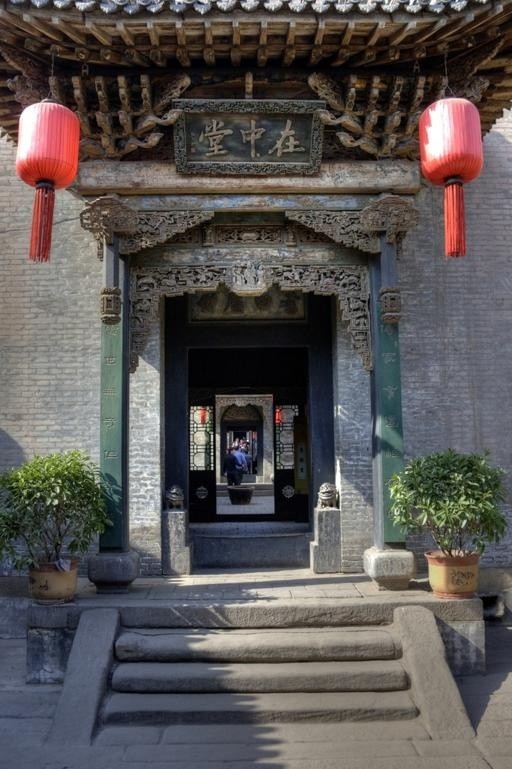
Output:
[386,444,510,599]
[0,449,116,605]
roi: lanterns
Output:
[418,97,484,185]
[15,98,81,189]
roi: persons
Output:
[223,436,251,486]
